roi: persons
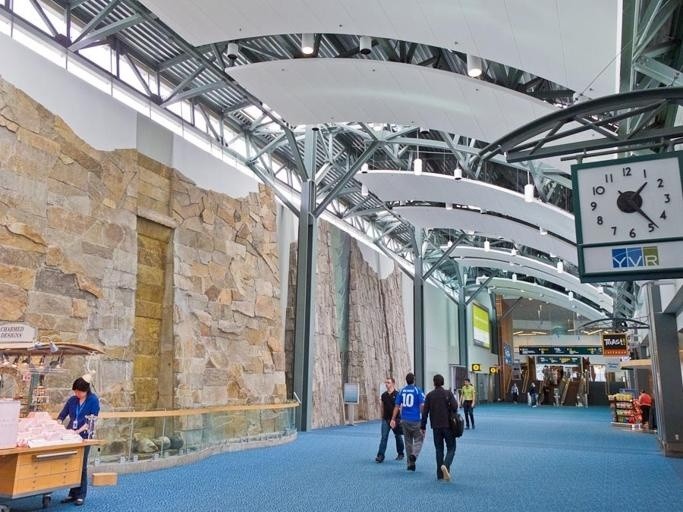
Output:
[510,382,519,404]
[55,376,100,507]
[419,373,458,482]
[376,376,403,462]
[459,378,475,430]
[389,372,428,471]
[529,381,536,407]
[638,389,651,428]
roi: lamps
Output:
[299,33,316,57]
[360,157,604,303]
[465,55,487,79]
[226,41,240,60]
[34,335,59,354]
[359,38,372,55]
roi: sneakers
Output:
[465,424,475,430]
[407,455,416,471]
[375,453,385,462]
[395,453,404,460]
[438,464,452,482]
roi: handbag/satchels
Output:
[449,413,465,438]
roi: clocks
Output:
[571,149,683,244]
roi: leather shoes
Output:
[60,496,75,503]
[76,498,84,506]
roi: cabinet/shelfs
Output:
[0,446,85,512]
